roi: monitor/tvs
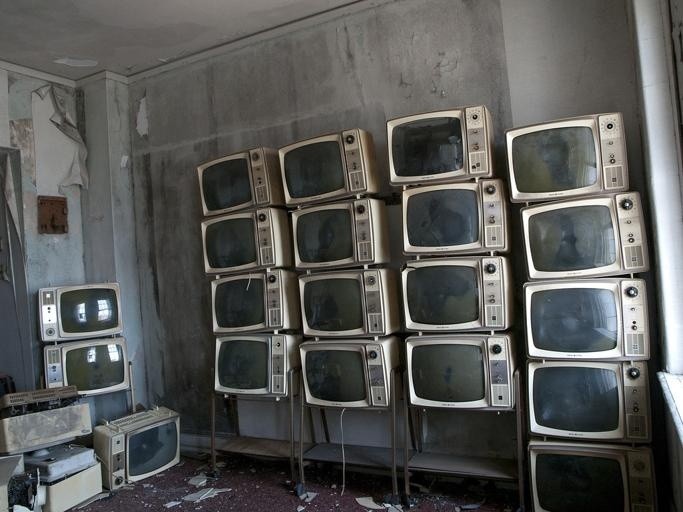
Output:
[386,104,496,191]
[298,267,400,342]
[214,334,303,401]
[299,336,399,407]
[38,280,123,341]
[402,179,511,261]
[400,256,513,335]
[196,146,283,217]
[93,403,180,491]
[504,112,629,207]
[201,207,291,280]
[404,332,518,414]
[278,128,381,209]
[528,439,660,512]
[522,277,651,366]
[44,334,130,398]
[526,359,652,445]
[291,197,391,275]
[211,269,300,334]
[519,191,650,279]
